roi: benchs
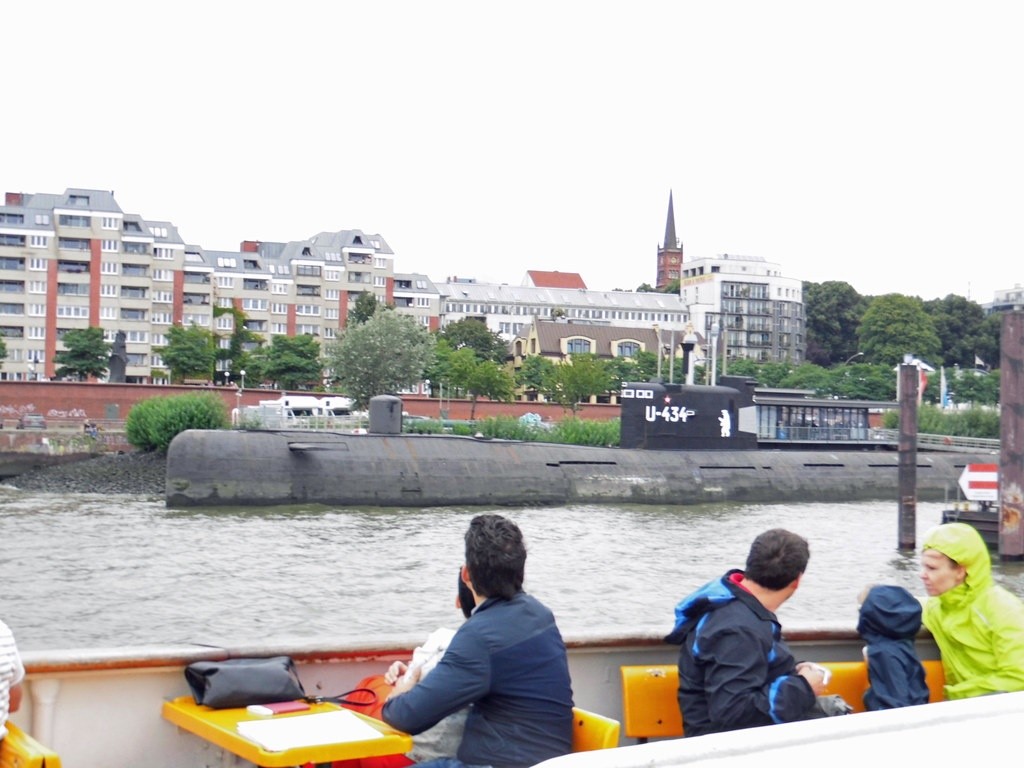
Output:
[814,661,944,712]
[345,675,620,768]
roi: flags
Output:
[920,368,928,405]
[941,369,948,408]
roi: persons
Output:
[678,527,852,739]
[384,561,475,767]
[382,513,574,768]
[856,522,1024,712]
[0,617,25,744]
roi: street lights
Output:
[224,371,230,386]
[844,352,865,367]
[239,369,246,388]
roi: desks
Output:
[161,696,415,768]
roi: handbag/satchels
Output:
[186,654,377,708]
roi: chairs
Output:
[619,665,684,745]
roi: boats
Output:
[0,619,1024,768]
[941,512,998,551]
[163,344,1001,511]
